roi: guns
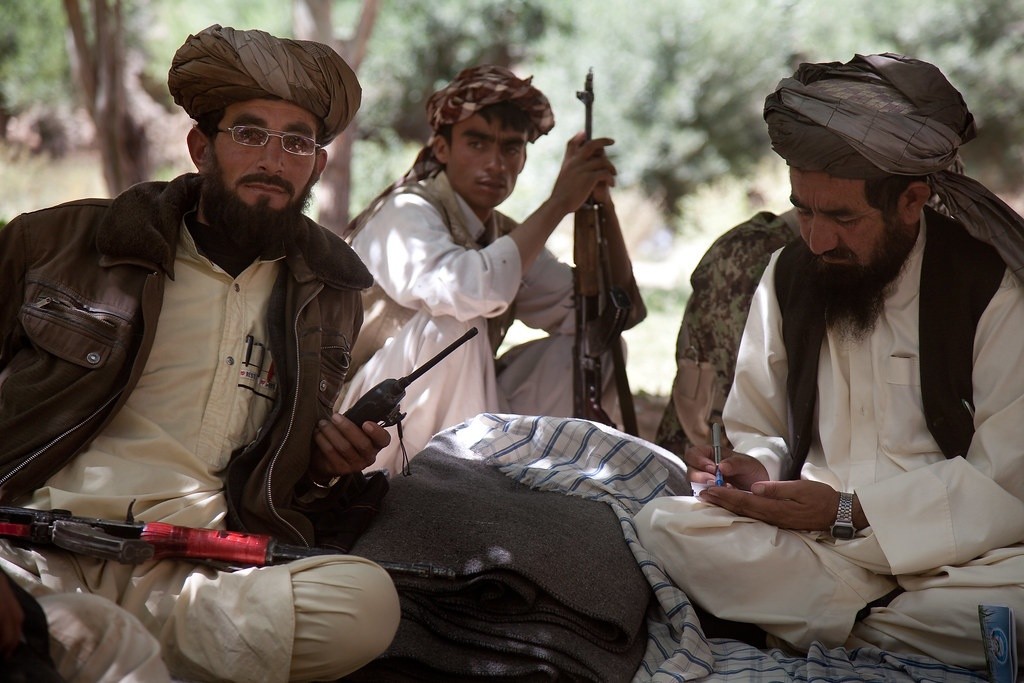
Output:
[571,67,648,446]
[1,497,463,608]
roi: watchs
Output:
[831,492,860,542]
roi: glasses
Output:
[216,124,323,156]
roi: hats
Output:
[425,64,556,144]
[168,23,362,148]
[763,51,1024,283]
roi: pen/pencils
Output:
[245,334,275,383]
[710,422,724,487]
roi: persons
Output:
[631,58,1024,662]
[2,565,175,683]
[333,65,649,471]
[1,25,401,683]
